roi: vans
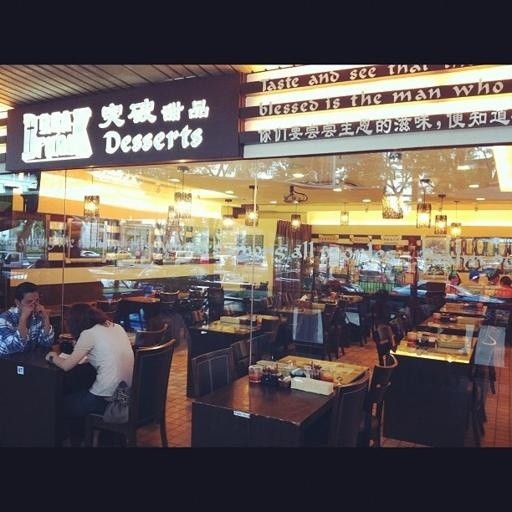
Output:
[167,250,197,262]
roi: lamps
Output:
[381,153,405,219]
[415,179,431,228]
[243,184,262,227]
[83,176,102,219]
[450,201,463,239]
[340,201,349,226]
[434,195,447,234]
[291,204,301,233]
[173,165,193,218]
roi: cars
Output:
[2,252,34,273]
[233,247,512,304]
[114,250,132,257]
[93,265,265,332]
[81,251,101,258]
[199,253,211,263]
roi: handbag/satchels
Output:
[102,380,131,425]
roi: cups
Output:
[433,312,441,323]
[475,302,483,311]
[320,368,334,382]
[406,331,417,348]
[278,381,290,388]
[329,291,336,304]
[248,365,263,383]
[51,345,62,355]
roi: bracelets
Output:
[49,356,54,363]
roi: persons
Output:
[441,271,512,327]
[46,302,134,447]
[0,282,56,356]
